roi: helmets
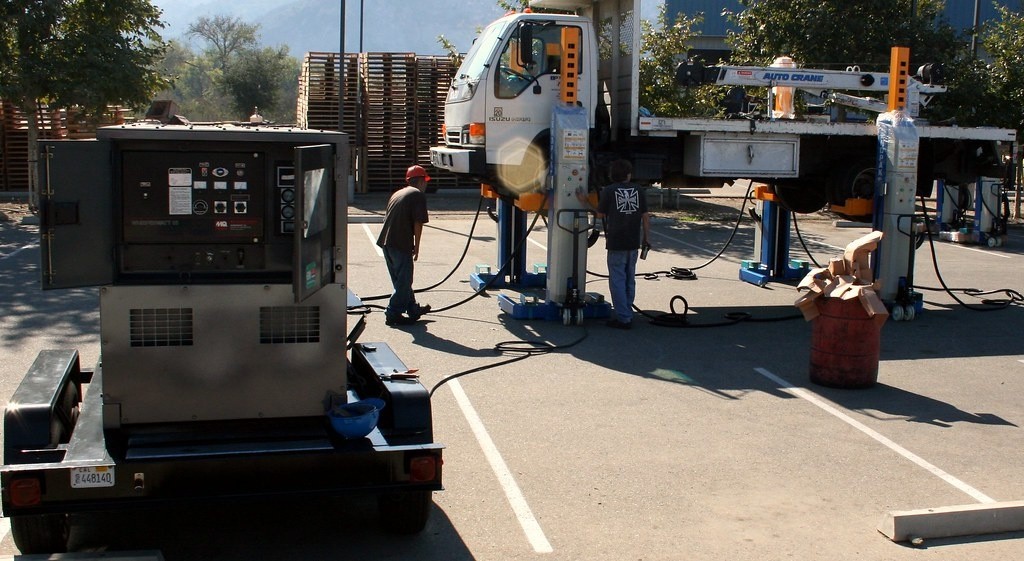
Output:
[405,165,431,182]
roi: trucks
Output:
[427,1,1017,194]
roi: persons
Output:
[576,160,651,327]
[377,166,431,325]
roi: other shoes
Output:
[606,319,632,330]
[408,303,431,321]
[385,313,416,326]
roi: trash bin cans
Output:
[805,291,883,388]
